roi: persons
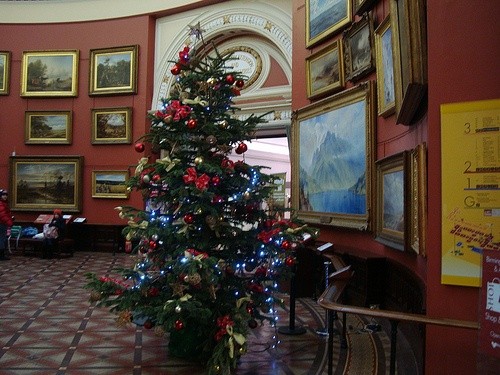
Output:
[45,208,66,259]
[0,189,15,261]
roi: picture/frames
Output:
[90,106,133,144]
[19,49,79,97]
[0,51,12,95]
[291,1,425,258]
[25,109,72,144]
[9,154,85,213]
[88,43,140,96]
[91,170,130,199]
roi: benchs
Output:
[7,238,74,259]
